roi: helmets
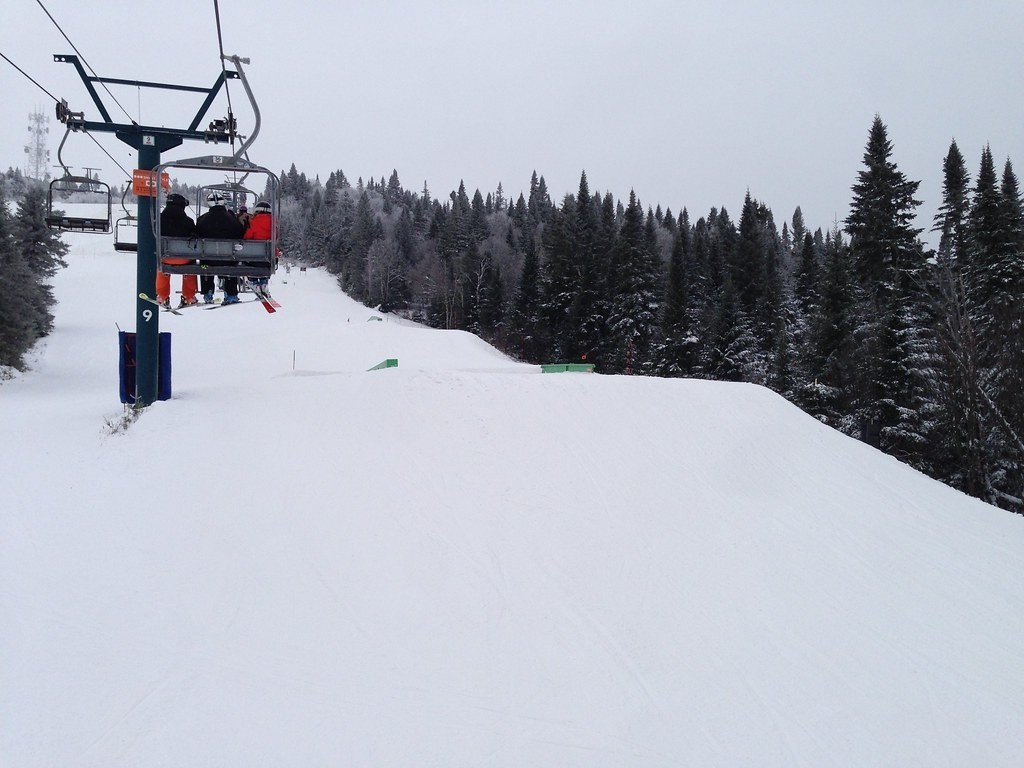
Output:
[207,193,224,206]
[167,194,186,208]
[240,206,247,212]
[254,201,272,214]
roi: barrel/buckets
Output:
[117,329,172,404]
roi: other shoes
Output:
[204,293,212,303]
[226,293,238,303]
[161,297,170,308]
[183,295,199,304]
[250,282,261,293]
[261,283,270,298]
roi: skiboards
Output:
[185,297,260,310]
[139,291,201,315]
[244,280,281,313]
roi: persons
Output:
[196,194,245,303]
[243,202,280,298]
[228,205,253,225]
[156,194,198,305]
